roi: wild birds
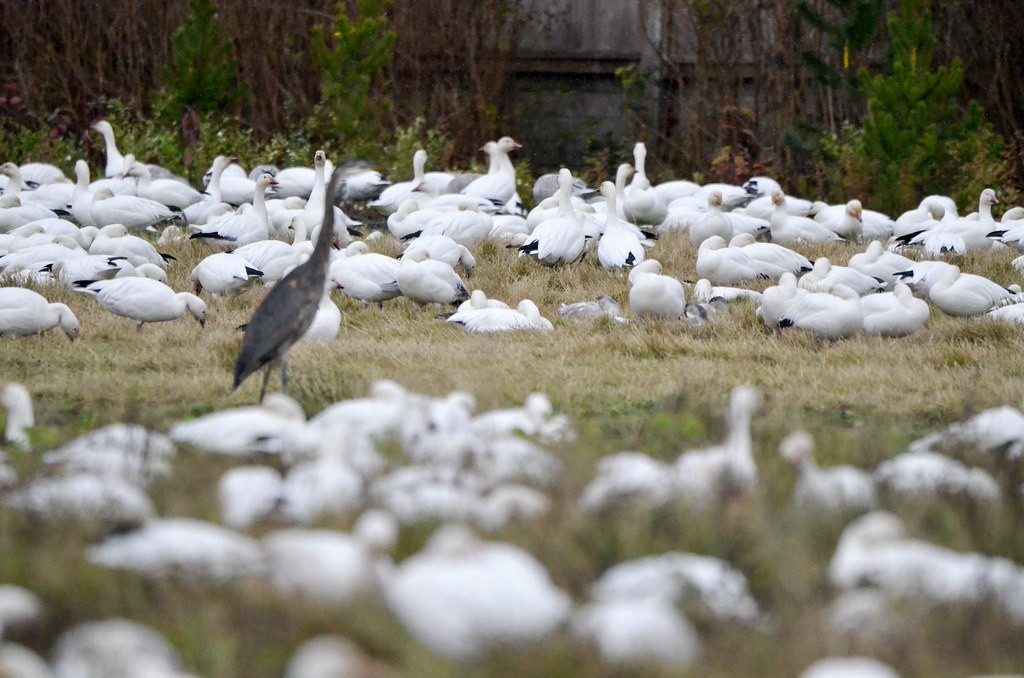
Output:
[0,376,1024,678]
[0,121,1024,403]
